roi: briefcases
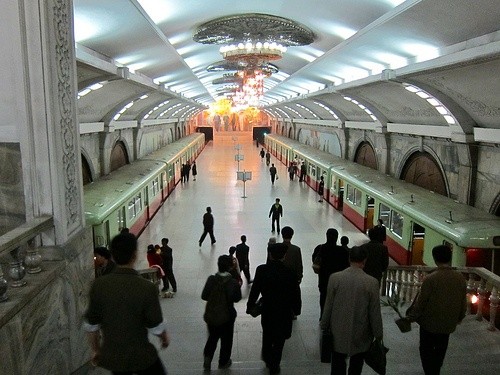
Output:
[320,328,333,363]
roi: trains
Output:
[263,132,500,324]
[83,128,206,251]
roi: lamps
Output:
[219,40,287,111]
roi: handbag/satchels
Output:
[365,337,390,375]
[250,297,265,317]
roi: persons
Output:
[266,151,270,164]
[260,148,265,158]
[267,237,277,261]
[81,233,168,375]
[299,162,306,182]
[405,245,467,375]
[311,228,350,321]
[320,245,383,375]
[92,247,115,277]
[228,246,243,287]
[359,228,389,290]
[374,219,386,244]
[147,238,163,293]
[160,238,177,293]
[269,163,277,185]
[198,206,216,247]
[235,235,254,284]
[318,176,325,202]
[180,160,197,183]
[267,226,304,320]
[288,161,297,182]
[201,255,243,375]
[269,198,283,235]
[246,243,302,375]
[340,236,351,262]
